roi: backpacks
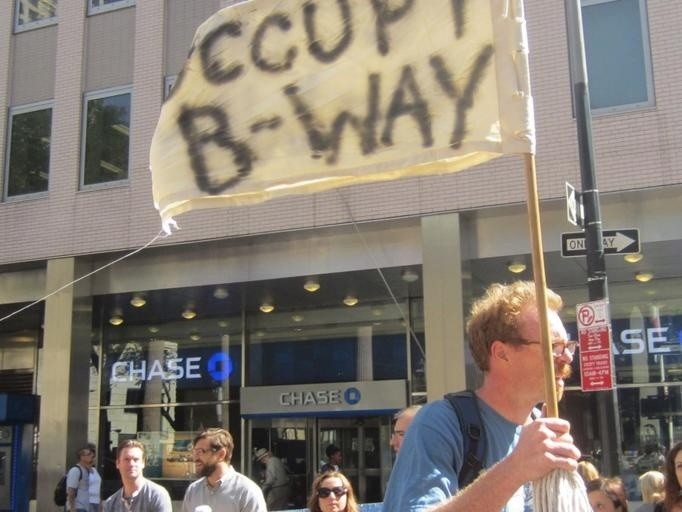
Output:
[54,466,82,508]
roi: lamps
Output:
[633,271,654,283]
[506,260,526,275]
[109,280,359,327]
[620,252,643,263]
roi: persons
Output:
[102,447,121,481]
[577,441,682,512]
[181,428,267,511]
[67,443,101,511]
[390,404,422,455]
[308,468,360,511]
[382,280,595,512]
[320,444,343,474]
[256,448,290,511]
[101,439,173,512]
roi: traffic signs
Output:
[559,226,641,258]
[565,178,586,232]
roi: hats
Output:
[255,448,270,462]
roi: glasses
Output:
[318,487,348,498]
[195,447,216,455]
[488,336,579,359]
